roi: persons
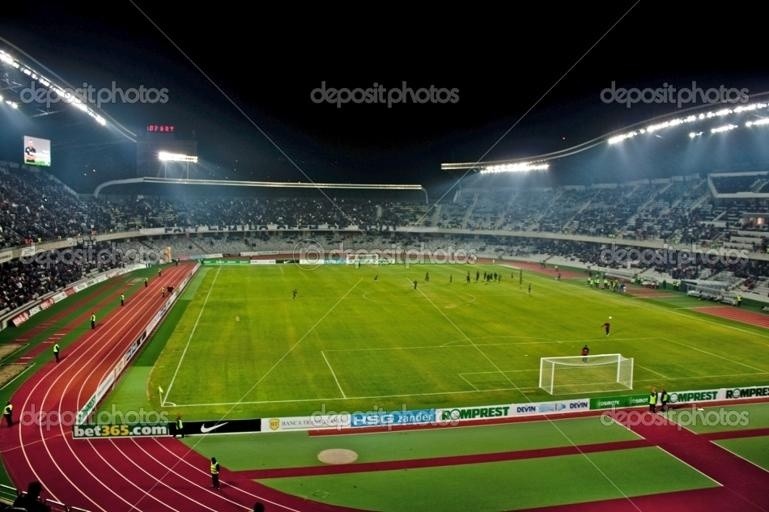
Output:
[54,342,60,362]
[25,139,37,162]
[253,502,265,512]
[4,400,13,427]
[210,457,220,491]
[0,161,769,315]
[172,415,184,440]
[90,312,96,328]
[581,344,589,363]
[13,481,51,512]
[648,392,657,412]
[660,390,668,412]
[601,323,610,336]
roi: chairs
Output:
[591,172,769,305]
[122,177,590,263]
